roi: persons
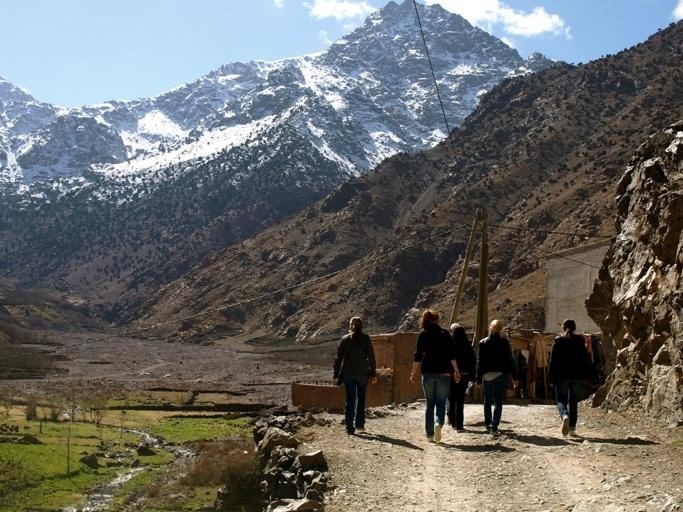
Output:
[447,326,476,432]
[450,323,461,336]
[548,319,589,436]
[475,318,519,436]
[410,310,461,442]
[334,317,378,434]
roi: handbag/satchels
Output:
[335,370,343,386]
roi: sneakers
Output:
[486,426,501,436]
[562,416,569,435]
[428,423,441,442]
[453,425,468,433]
[347,423,365,435]
[567,434,583,440]
